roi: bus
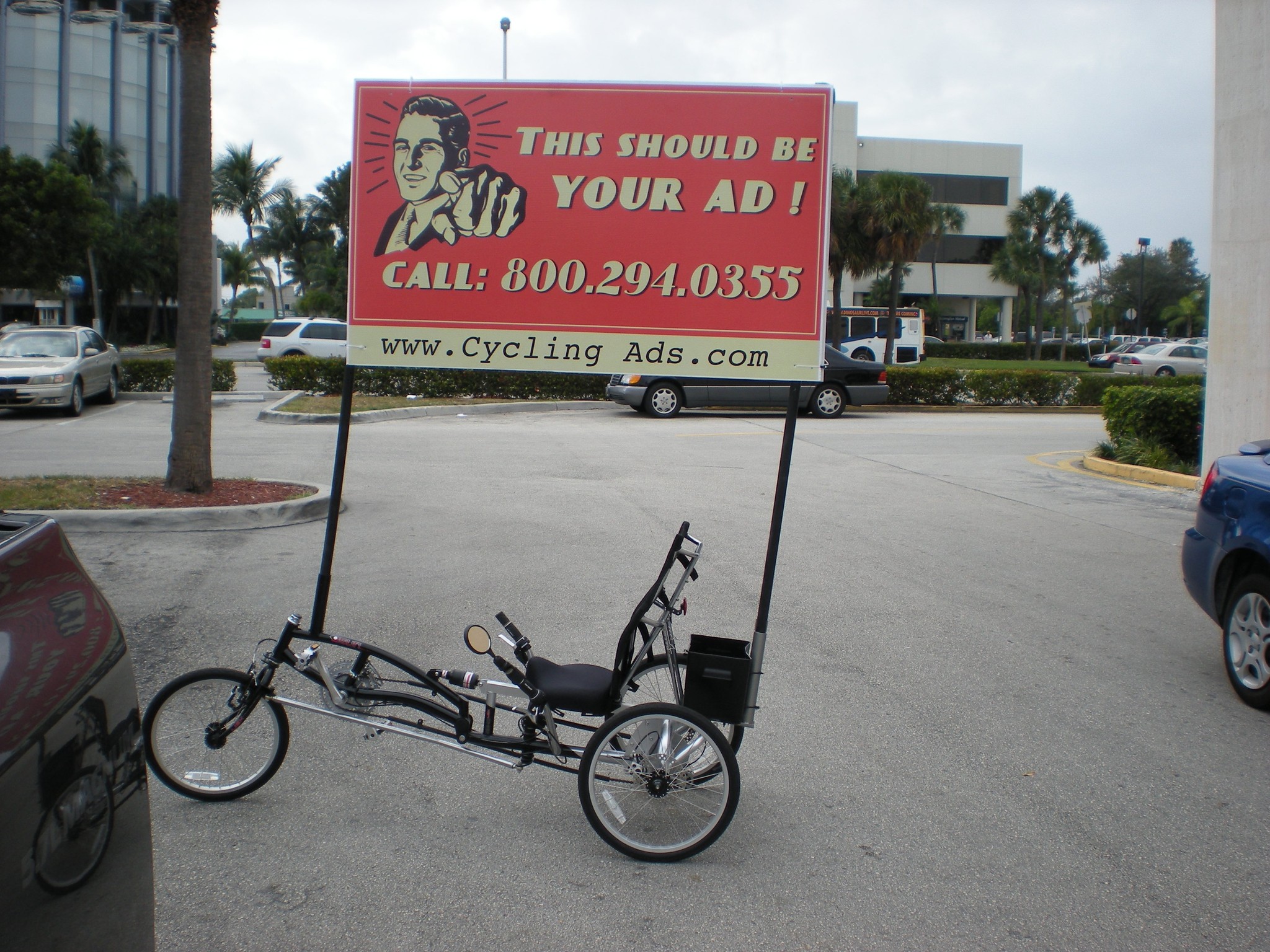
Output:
[825,305,927,364]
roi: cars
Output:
[1181,436,1270,711]
[605,343,890,418]
[924,335,945,343]
[977,330,1208,369]
[1113,341,1208,379]
[0,320,35,342]
[0,325,123,418]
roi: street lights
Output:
[1137,237,1151,334]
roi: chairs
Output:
[513,520,703,709]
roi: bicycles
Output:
[141,356,800,866]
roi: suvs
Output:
[255,316,348,364]
